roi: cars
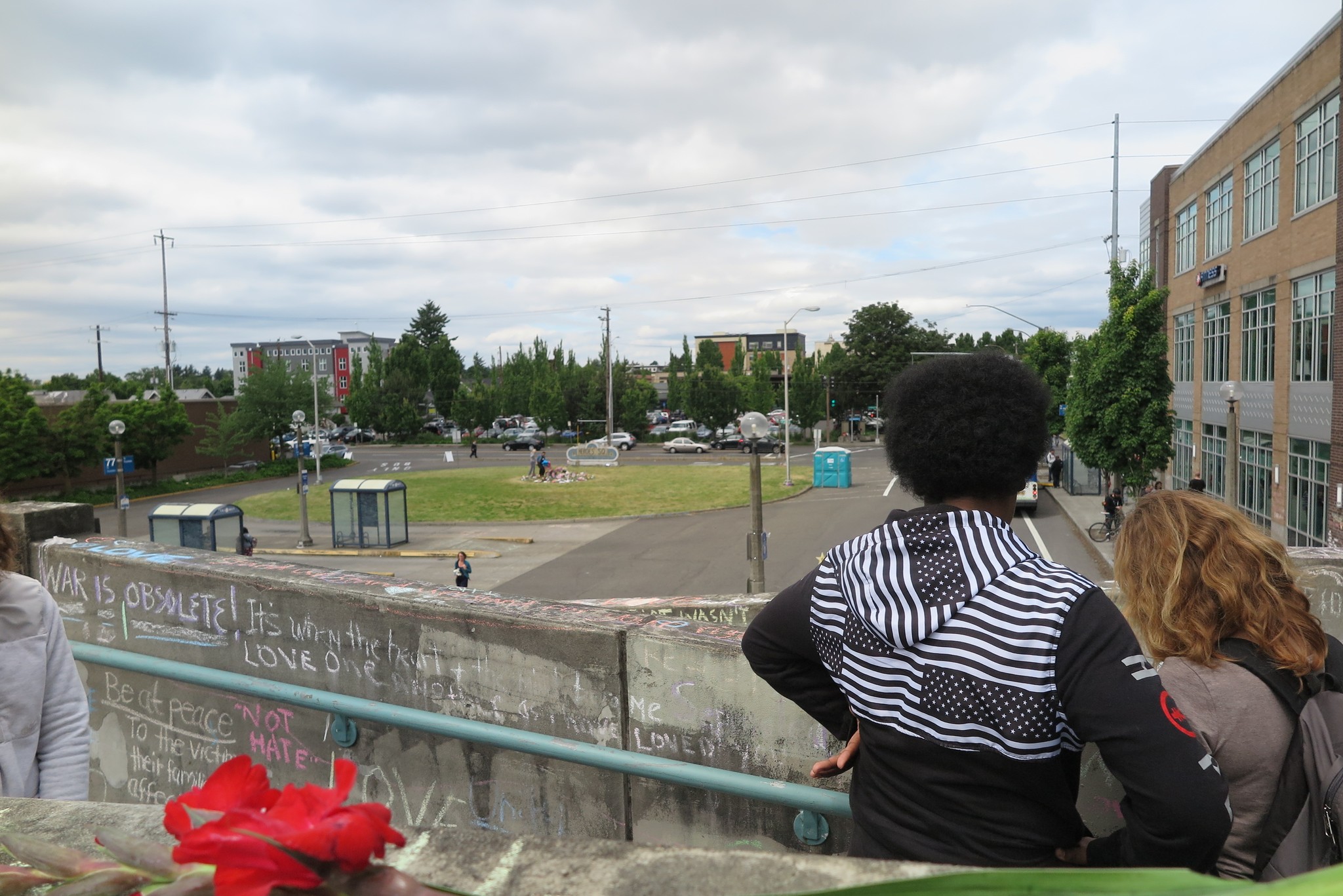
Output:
[646,410,668,439]
[662,437,712,453]
[502,436,544,451]
[271,427,376,459]
[561,431,585,437]
[424,418,457,434]
[670,413,688,422]
[539,426,560,436]
[849,413,885,430]
[460,415,543,438]
[716,410,790,454]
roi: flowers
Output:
[159,756,406,896]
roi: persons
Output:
[0,496,90,800]
[1104,488,1123,542]
[528,449,546,478]
[236,527,253,556]
[1140,472,1207,497]
[469,441,478,458]
[1076,490,1343,883]
[1047,449,1063,488]
[200,526,212,547]
[741,348,1233,873]
[453,552,471,587]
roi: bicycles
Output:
[1087,508,1126,542]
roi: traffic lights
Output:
[871,414,876,418]
[829,395,835,407]
[821,374,836,390]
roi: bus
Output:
[1017,470,1039,514]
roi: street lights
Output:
[782,305,820,486]
[739,411,769,592]
[291,333,323,484]
[292,410,314,547]
[1219,381,1245,509]
[108,419,129,536]
[963,303,1049,354]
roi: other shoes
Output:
[1107,538,1110,542]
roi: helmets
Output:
[1113,488,1121,495]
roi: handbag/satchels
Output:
[250,537,257,548]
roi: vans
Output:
[669,419,698,434]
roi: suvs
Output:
[588,433,637,452]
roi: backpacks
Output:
[1207,633,1343,877]
[1046,454,1055,464]
